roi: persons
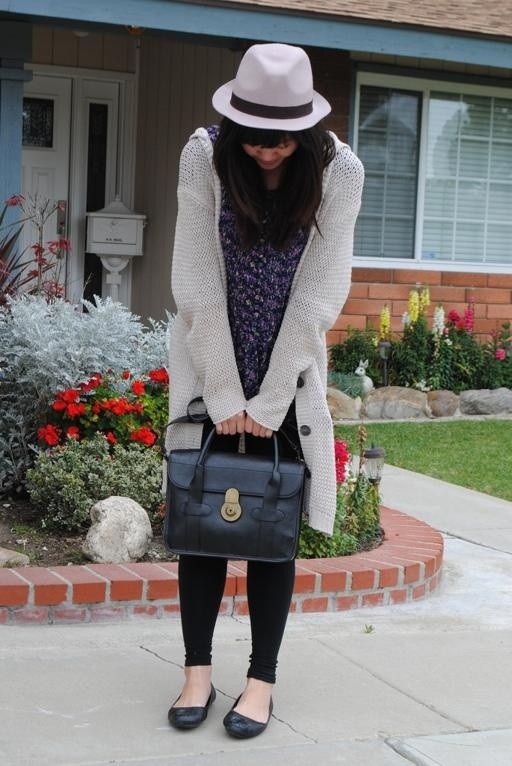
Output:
[161,43,365,738]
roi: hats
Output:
[209,41,336,135]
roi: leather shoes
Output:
[223,689,275,743]
[167,682,217,732]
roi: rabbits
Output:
[354,359,373,393]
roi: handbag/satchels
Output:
[162,390,310,565]
